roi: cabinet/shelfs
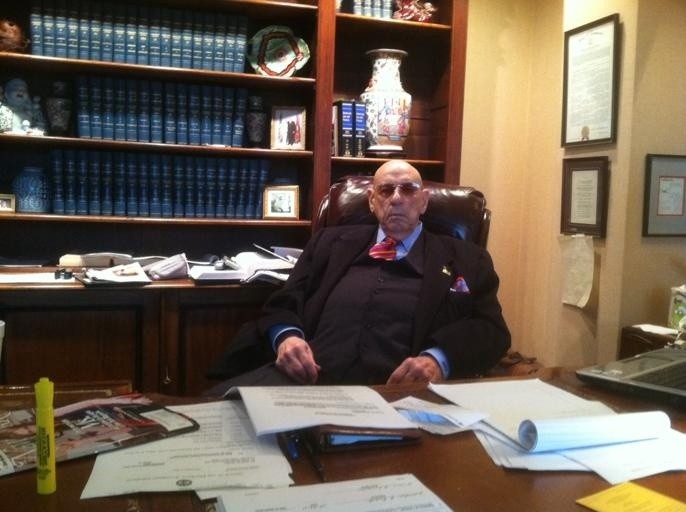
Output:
[0,0,468,397]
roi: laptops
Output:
[575,348,686,405]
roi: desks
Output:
[0,363,686,512]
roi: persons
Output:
[200,162,512,394]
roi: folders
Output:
[312,422,423,451]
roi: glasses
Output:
[373,181,421,196]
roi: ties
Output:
[367,235,400,262]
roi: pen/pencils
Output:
[280,431,303,459]
[34,378,56,495]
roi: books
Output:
[337,0,395,21]
[330,99,372,158]
[0,392,198,477]
[30,5,248,73]
[51,147,271,219]
[77,76,249,146]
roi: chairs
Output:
[311,174,491,249]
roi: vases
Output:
[245,96,268,148]
[46,82,74,137]
[10,166,53,214]
[359,48,412,157]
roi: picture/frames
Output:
[560,156,609,239]
[560,13,620,149]
[270,105,306,151]
[262,183,300,222]
[643,154,686,238]
